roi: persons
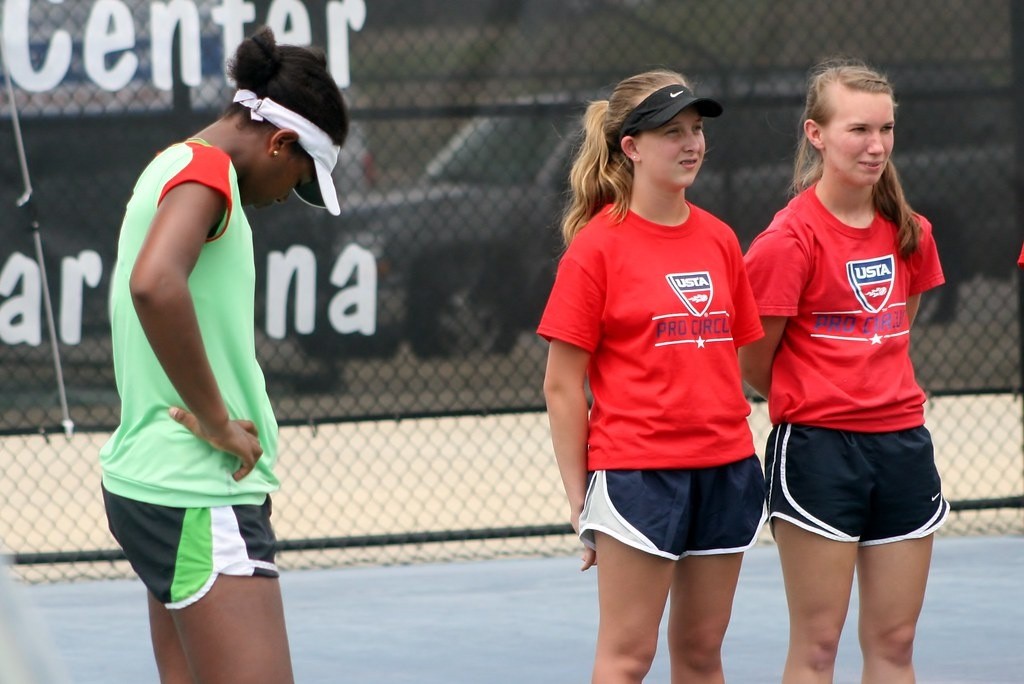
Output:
[738,63,951,684]
[537,70,768,684]
[98,28,349,684]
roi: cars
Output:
[296,81,964,364]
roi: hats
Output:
[233,89,340,215]
[620,84,724,143]
